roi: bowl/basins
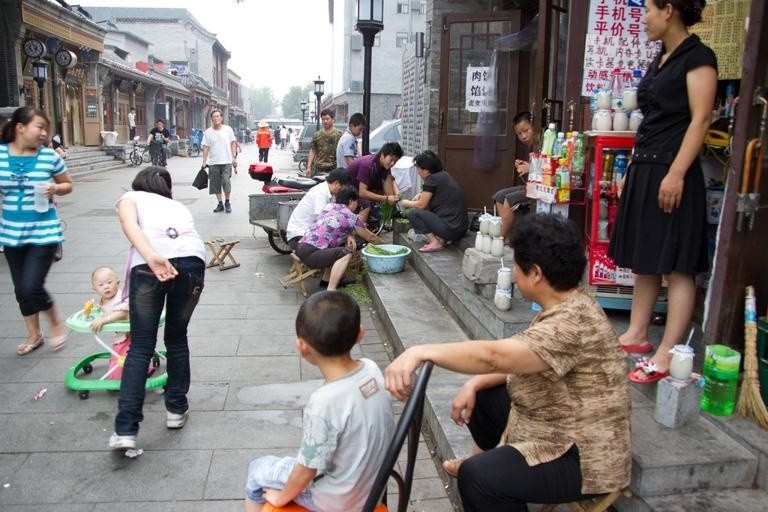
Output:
[363,243,412,274]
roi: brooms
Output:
[737,286,768,431]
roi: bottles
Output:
[608,65,643,112]
[587,86,598,112]
[527,121,585,185]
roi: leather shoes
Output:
[632,147,677,166]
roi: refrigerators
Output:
[585,136,672,316]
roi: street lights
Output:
[31,56,49,112]
[300,98,307,125]
[351,0,388,158]
[311,72,326,132]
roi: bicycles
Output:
[296,154,315,172]
[128,135,165,168]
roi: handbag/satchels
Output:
[191,163,210,191]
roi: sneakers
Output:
[320,277,358,288]
[225,203,232,212]
[165,406,190,429]
[109,433,137,450]
[214,205,224,212]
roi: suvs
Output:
[291,119,347,163]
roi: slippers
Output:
[619,338,654,354]
[418,234,448,253]
[628,356,671,384]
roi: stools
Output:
[542,474,638,510]
[276,250,323,299]
[202,238,242,272]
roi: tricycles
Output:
[187,125,203,158]
[247,182,384,263]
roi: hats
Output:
[258,121,269,128]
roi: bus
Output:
[252,116,305,136]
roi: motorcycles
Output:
[244,159,330,193]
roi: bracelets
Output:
[232,156,237,159]
[385,196,389,205]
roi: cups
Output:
[592,91,645,132]
[475,216,504,257]
[495,265,512,311]
[668,343,695,382]
[33,181,51,213]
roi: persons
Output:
[293,184,383,291]
[285,168,358,279]
[399,148,469,253]
[335,113,367,168]
[105,165,208,451]
[88,267,158,380]
[379,208,634,511]
[145,118,171,169]
[346,140,404,214]
[199,109,238,214]
[491,110,540,236]
[303,107,342,178]
[240,290,396,511]
[0,106,74,355]
[169,125,180,139]
[245,121,292,164]
[127,107,137,139]
[603,0,722,386]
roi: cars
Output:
[355,114,402,154]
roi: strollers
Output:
[64,304,171,401]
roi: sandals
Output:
[47,326,71,351]
[17,333,43,356]
[441,457,467,477]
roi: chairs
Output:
[355,359,435,508]
